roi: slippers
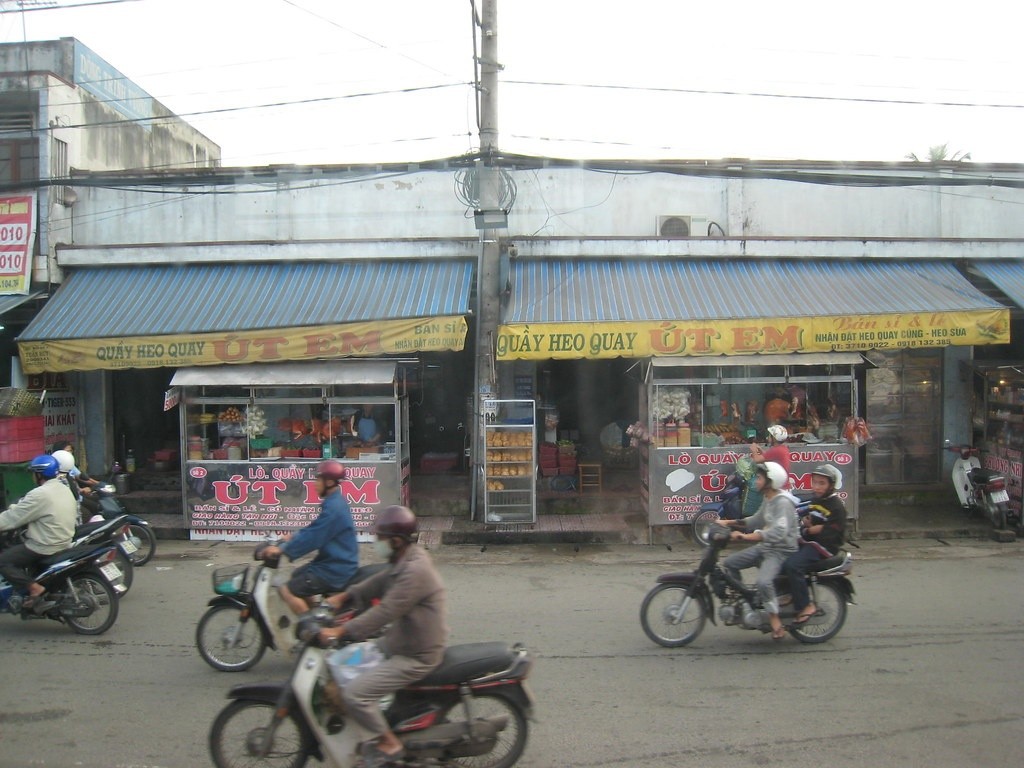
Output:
[772,626,785,640]
[24,591,49,607]
[793,610,818,624]
[364,749,407,768]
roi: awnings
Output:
[496,261,1011,361]
[15,260,475,375]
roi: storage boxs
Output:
[346,446,380,457]
[0,415,44,462]
[540,442,578,476]
[420,453,460,471]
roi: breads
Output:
[217,408,245,421]
[486,430,534,489]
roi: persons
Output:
[0,455,77,610]
[751,425,790,490]
[52,450,83,528]
[716,462,799,640]
[320,504,450,768]
[264,459,358,654]
[53,441,99,496]
[778,464,847,626]
[351,404,384,445]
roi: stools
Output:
[578,461,602,491]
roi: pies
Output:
[703,424,737,433]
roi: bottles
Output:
[125,449,136,472]
[227,443,242,460]
[652,419,691,447]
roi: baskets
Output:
[303,450,321,458]
[282,450,303,457]
[539,441,577,476]
[250,438,274,449]
[214,563,248,593]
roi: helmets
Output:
[768,425,788,442]
[28,455,59,476]
[811,464,836,491]
[757,462,787,491]
[52,450,74,472]
[375,505,419,543]
[315,461,345,479]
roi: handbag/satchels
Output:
[327,642,396,714]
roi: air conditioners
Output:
[656,214,692,237]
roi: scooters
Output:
[80,462,156,567]
[71,514,139,606]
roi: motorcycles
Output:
[942,438,1011,528]
[207,602,534,762]
[0,539,122,635]
[194,536,391,673]
[638,514,853,648]
